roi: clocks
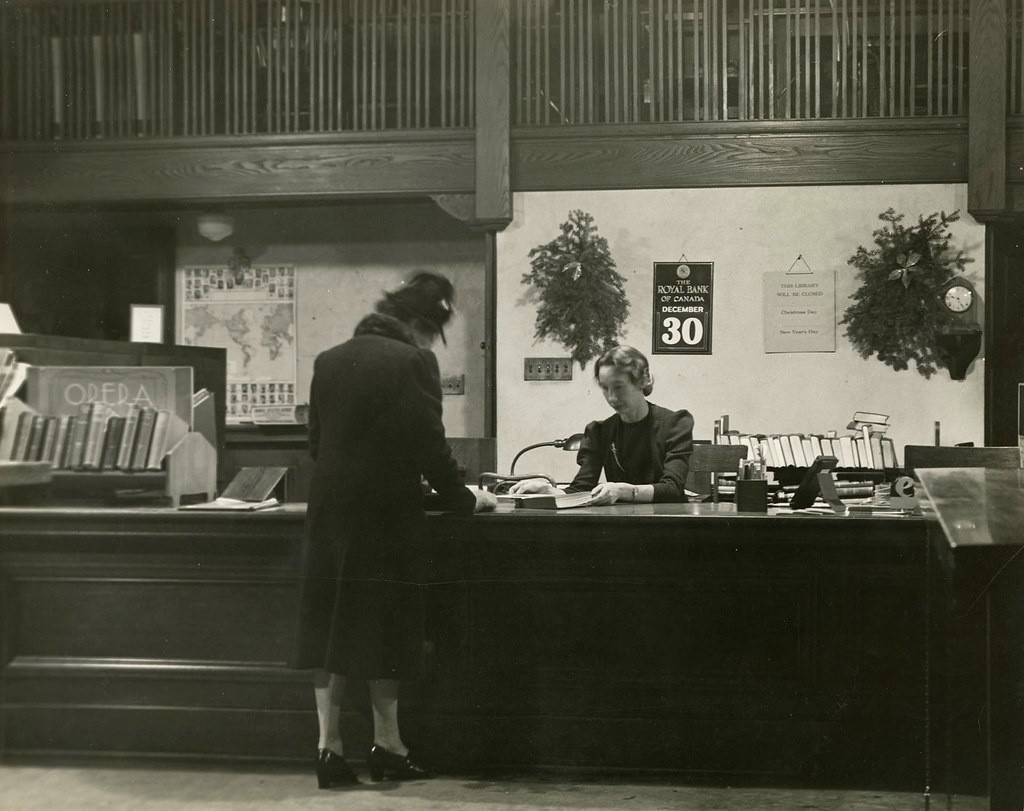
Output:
[938,274,982,380]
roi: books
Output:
[177,496,279,511]
[0,396,190,471]
[496,490,593,509]
[712,410,898,502]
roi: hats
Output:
[382,273,455,347]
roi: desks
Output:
[0,497,1023,796]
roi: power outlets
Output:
[524,358,572,381]
[441,373,465,396]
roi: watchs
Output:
[630,485,638,503]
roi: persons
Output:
[508,346,694,506]
[290,270,497,789]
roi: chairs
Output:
[684,444,748,503]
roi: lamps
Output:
[197,207,234,243]
[510,432,584,476]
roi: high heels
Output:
[316,748,359,788]
[367,744,438,782]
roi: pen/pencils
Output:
[739,457,767,481]
